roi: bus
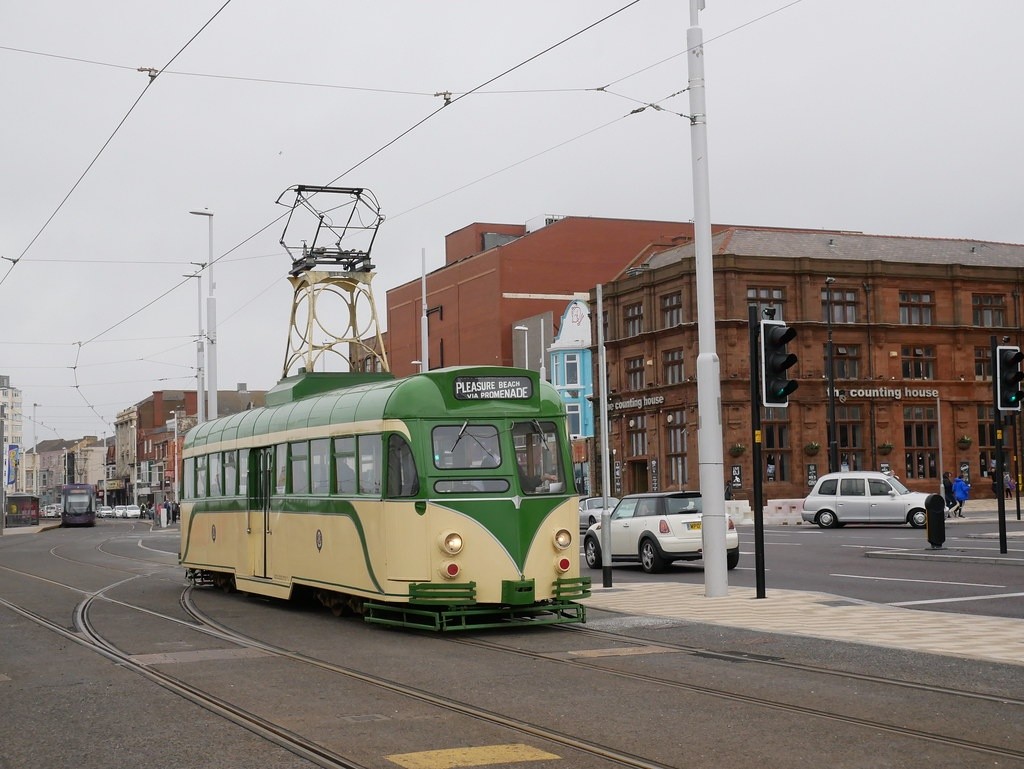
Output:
[177,184,593,635]
[61,484,97,526]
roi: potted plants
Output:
[804,440,821,456]
[729,443,745,456]
[877,441,896,454]
[958,434,972,450]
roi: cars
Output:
[98,506,114,519]
[579,497,621,529]
[112,506,126,518]
[801,470,930,528]
[122,506,141,519]
[40,509,44,517]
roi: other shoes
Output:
[1005,498,1008,500]
[959,515,964,517]
[1011,497,1013,500]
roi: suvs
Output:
[584,491,739,573]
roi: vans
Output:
[56,504,61,516]
[44,505,59,518]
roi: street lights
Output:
[169,411,178,505]
[826,278,839,473]
[75,441,79,483]
[102,432,107,506]
[130,425,138,506]
[190,207,217,421]
[62,447,67,485]
[33,403,42,497]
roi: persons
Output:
[725,480,735,500]
[139,499,178,524]
[988,467,998,499]
[952,475,971,518]
[1004,475,1014,499]
[943,472,957,518]
[481,436,558,493]
[111,503,117,518]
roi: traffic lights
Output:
[762,319,800,408]
[996,345,1024,411]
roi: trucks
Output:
[96,498,104,516]
